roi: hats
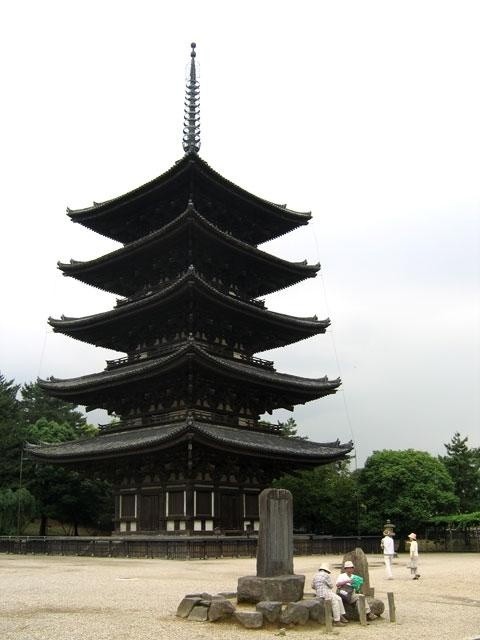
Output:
[318,563,331,573]
[383,530,390,535]
[407,533,416,539]
[344,561,354,568]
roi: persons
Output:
[336,560,378,626]
[311,563,349,627]
[380,530,396,580]
[405,533,420,580]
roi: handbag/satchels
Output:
[336,585,354,601]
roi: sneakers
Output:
[334,616,349,626]
[368,613,378,619]
